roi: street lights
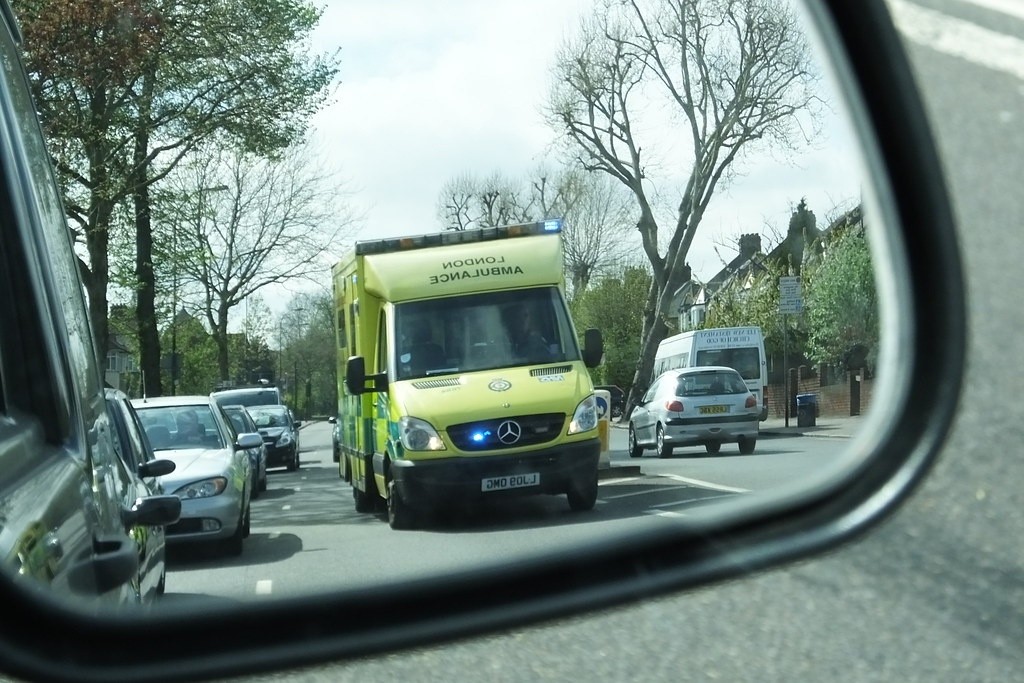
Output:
[279,307,304,382]
[170,184,231,397]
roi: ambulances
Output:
[330,219,603,531]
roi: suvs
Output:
[102,385,183,608]
[593,385,626,417]
[0,2,141,614]
[207,378,280,409]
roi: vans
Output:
[648,326,769,421]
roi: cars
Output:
[329,417,342,461]
[222,406,269,500]
[246,405,302,471]
[131,396,263,557]
[628,366,759,460]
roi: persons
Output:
[168,412,200,445]
[503,305,549,358]
[710,376,725,390]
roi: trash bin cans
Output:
[796,394,818,428]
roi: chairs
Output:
[403,318,446,377]
[710,382,724,393]
[148,426,173,451]
